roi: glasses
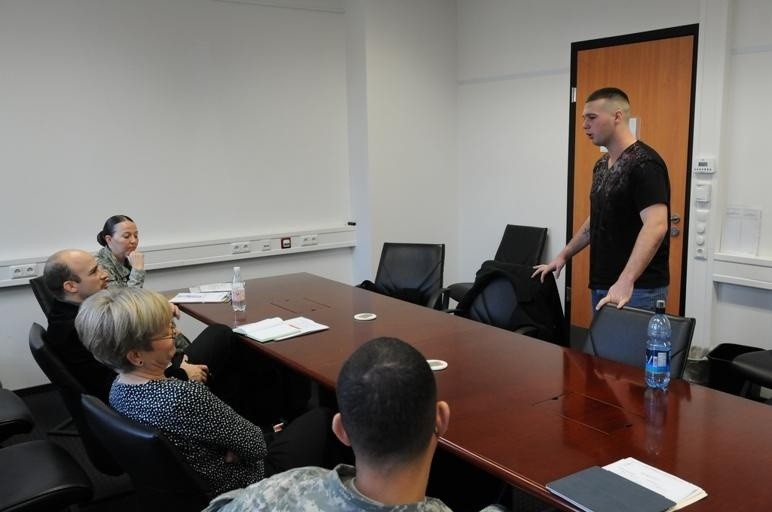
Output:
[132,321,177,350]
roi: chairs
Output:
[460,275,539,334]
[1,382,33,439]
[448,225,546,303]
[30,322,118,415]
[582,303,694,379]
[0,440,94,511]
[72,393,206,509]
[28,274,56,320]
[352,242,446,308]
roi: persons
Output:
[203,335,505,512]
[92,215,145,288]
[44,250,305,383]
[74,287,332,499]
[531,87,671,318]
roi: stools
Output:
[730,346,772,408]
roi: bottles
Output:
[232,267,246,312]
[643,300,672,388]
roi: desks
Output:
[155,270,771,512]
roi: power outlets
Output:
[260,240,271,249]
[231,242,252,252]
[9,264,37,277]
[301,234,317,246]
[281,237,291,248]
